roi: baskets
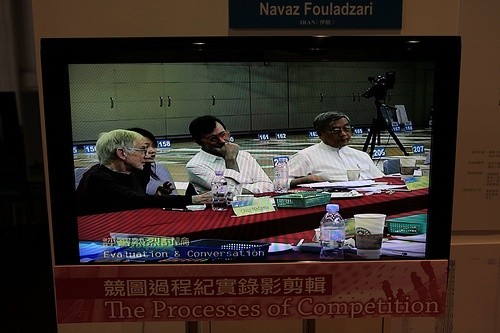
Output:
[276,191,331,208]
[386,213,427,236]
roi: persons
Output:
[184,115,275,194]
[74,129,213,216]
[273,112,386,191]
[125,126,179,195]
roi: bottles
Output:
[211,171,228,211]
[320,204,345,259]
[275,158,289,194]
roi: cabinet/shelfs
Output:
[70,63,377,145]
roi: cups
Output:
[237,195,254,202]
[347,169,361,181]
[354,213,387,259]
[400,158,416,179]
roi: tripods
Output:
[361,99,410,162]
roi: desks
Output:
[78,177,428,244]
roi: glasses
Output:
[207,130,227,144]
[128,146,149,157]
[329,126,353,134]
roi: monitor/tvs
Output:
[41,35,462,266]
